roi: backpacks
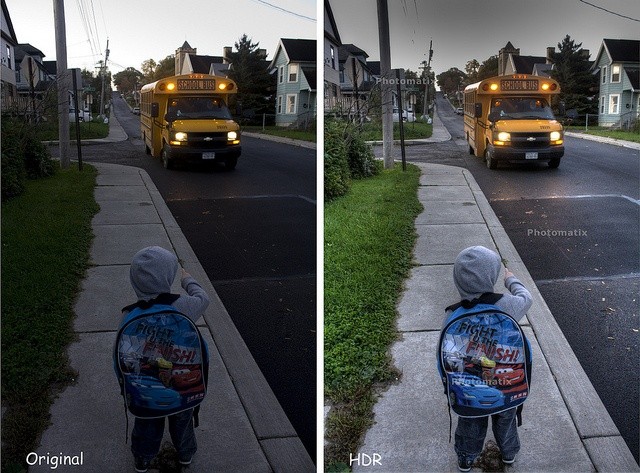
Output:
[113,294,209,443]
[435,291,533,442]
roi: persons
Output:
[438,245,534,472]
[114,245,209,468]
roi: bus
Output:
[464,73,564,168]
[140,72,242,168]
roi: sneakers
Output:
[458,454,475,471]
[179,455,193,464]
[502,453,517,463]
[133,454,150,472]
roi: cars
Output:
[68,107,92,124]
[393,108,417,123]
